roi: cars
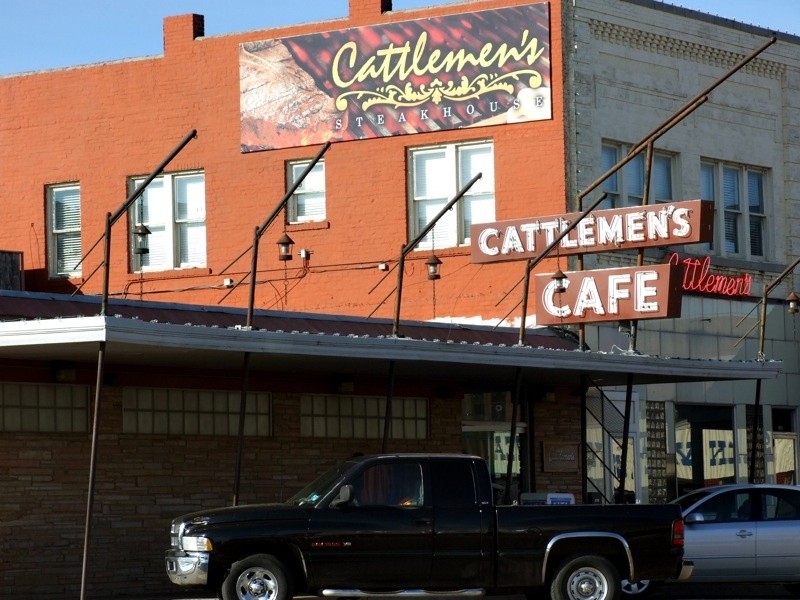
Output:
[620,482,800,597]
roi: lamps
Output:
[785,291,799,313]
[551,270,568,293]
[276,233,296,263]
[425,255,444,281]
[133,225,151,254]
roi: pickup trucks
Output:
[166,452,687,600]
